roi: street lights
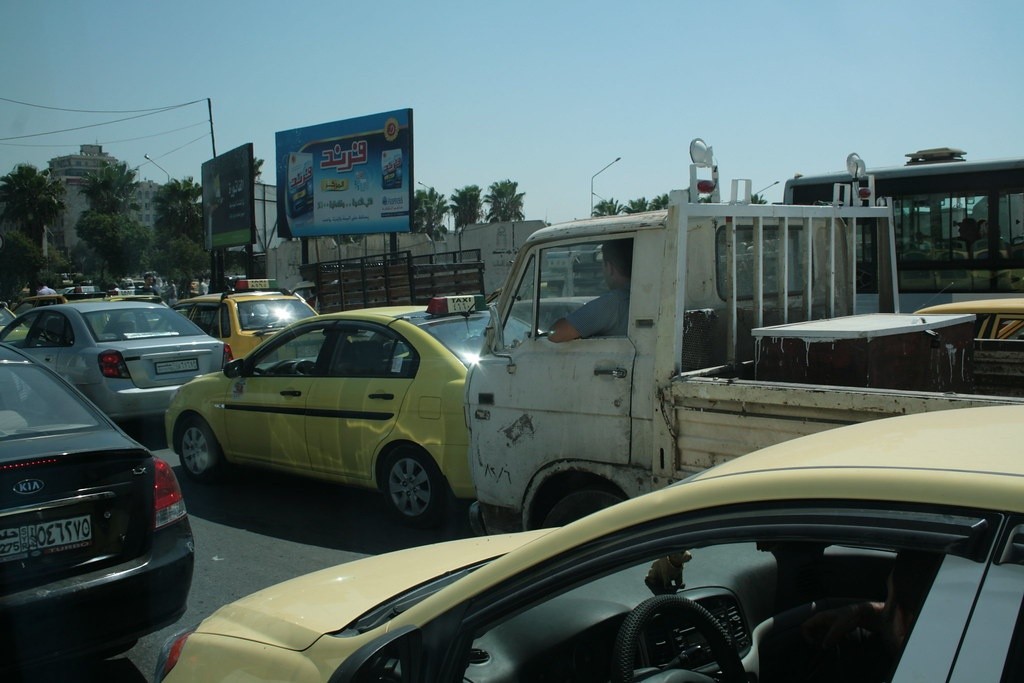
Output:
[591,157,622,216]
[419,181,451,231]
[754,180,780,197]
[144,155,169,183]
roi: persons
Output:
[142,272,160,296]
[168,280,177,306]
[198,278,208,295]
[548,238,633,343]
[36,282,56,296]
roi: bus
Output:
[783,146,1024,320]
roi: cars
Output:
[906,299,1024,342]
[147,401,1021,683]
[492,296,602,344]
[0,278,237,425]
[0,342,194,683]
[169,277,336,372]
[165,291,552,526]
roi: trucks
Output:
[460,137,1023,534]
